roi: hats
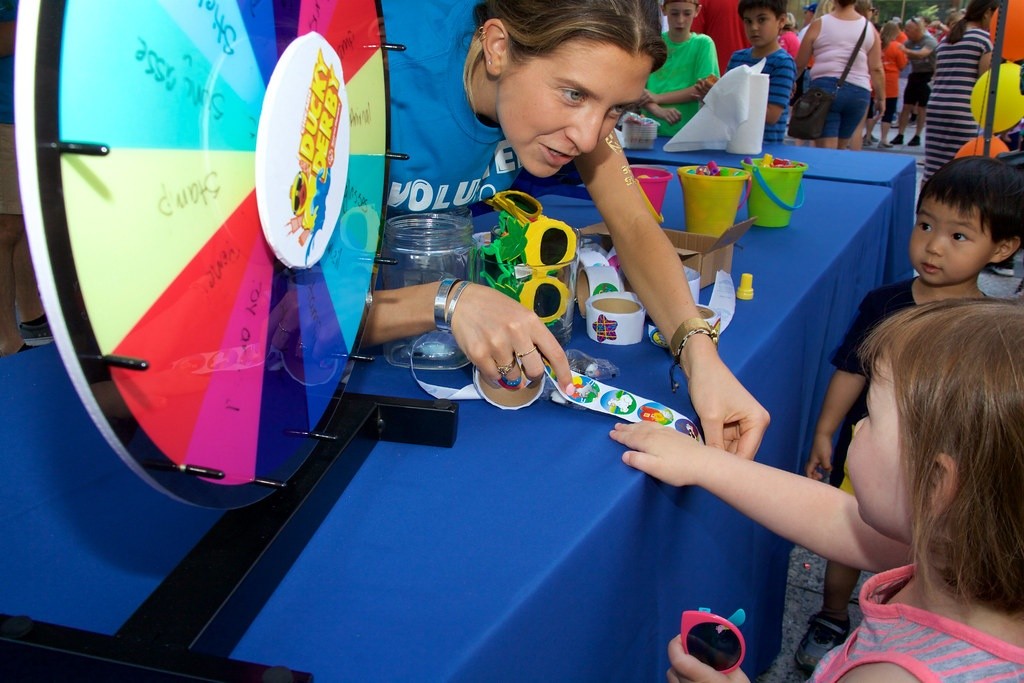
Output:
[892,17,902,23]
[664,0,698,6]
[803,3,818,13]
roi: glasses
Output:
[480,190,576,324]
[681,605,746,672]
[869,8,877,13]
[911,15,919,26]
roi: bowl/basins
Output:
[622,122,658,149]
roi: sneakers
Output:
[795,611,852,673]
[890,135,904,145]
[908,136,920,146]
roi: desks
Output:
[620,129,918,288]
[0,157,894,683]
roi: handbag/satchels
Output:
[788,91,833,140]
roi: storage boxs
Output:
[575,214,759,289]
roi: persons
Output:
[784,0,1024,281]
[362,0,774,465]
[795,154,1024,672]
[0,1,56,358]
[73,254,343,421]
[691,0,798,148]
[636,0,721,135]
[609,296,1023,683]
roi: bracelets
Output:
[433,277,470,334]
[668,319,721,395]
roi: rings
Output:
[279,322,293,335]
[514,345,537,358]
[297,339,312,352]
[497,355,516,376]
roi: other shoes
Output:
[863,134,879,142]
[891,121,917,128]
[862,138,873,147]
[19,320,55,346]
[987,262,1015,278]
[878,142,893,149]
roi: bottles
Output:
[381,212,479,370]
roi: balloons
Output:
[970,62,1024,135]
[954,135,1009,159]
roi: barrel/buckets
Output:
[741,158,808,227]
[677,166,752,237]
[629,167,674,225]
